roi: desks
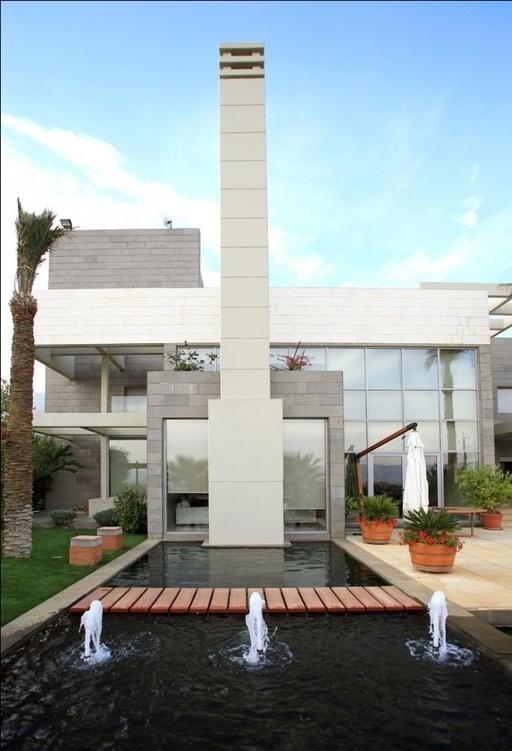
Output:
[429,505,488,537]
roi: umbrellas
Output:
[402,430,431,524]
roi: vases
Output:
[359,520,395,545]
[406,540,457,573]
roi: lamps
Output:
[60,218,73,230]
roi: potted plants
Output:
[454,461,512,530]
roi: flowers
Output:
[354,494,399,530]
[398,504,466,553]
